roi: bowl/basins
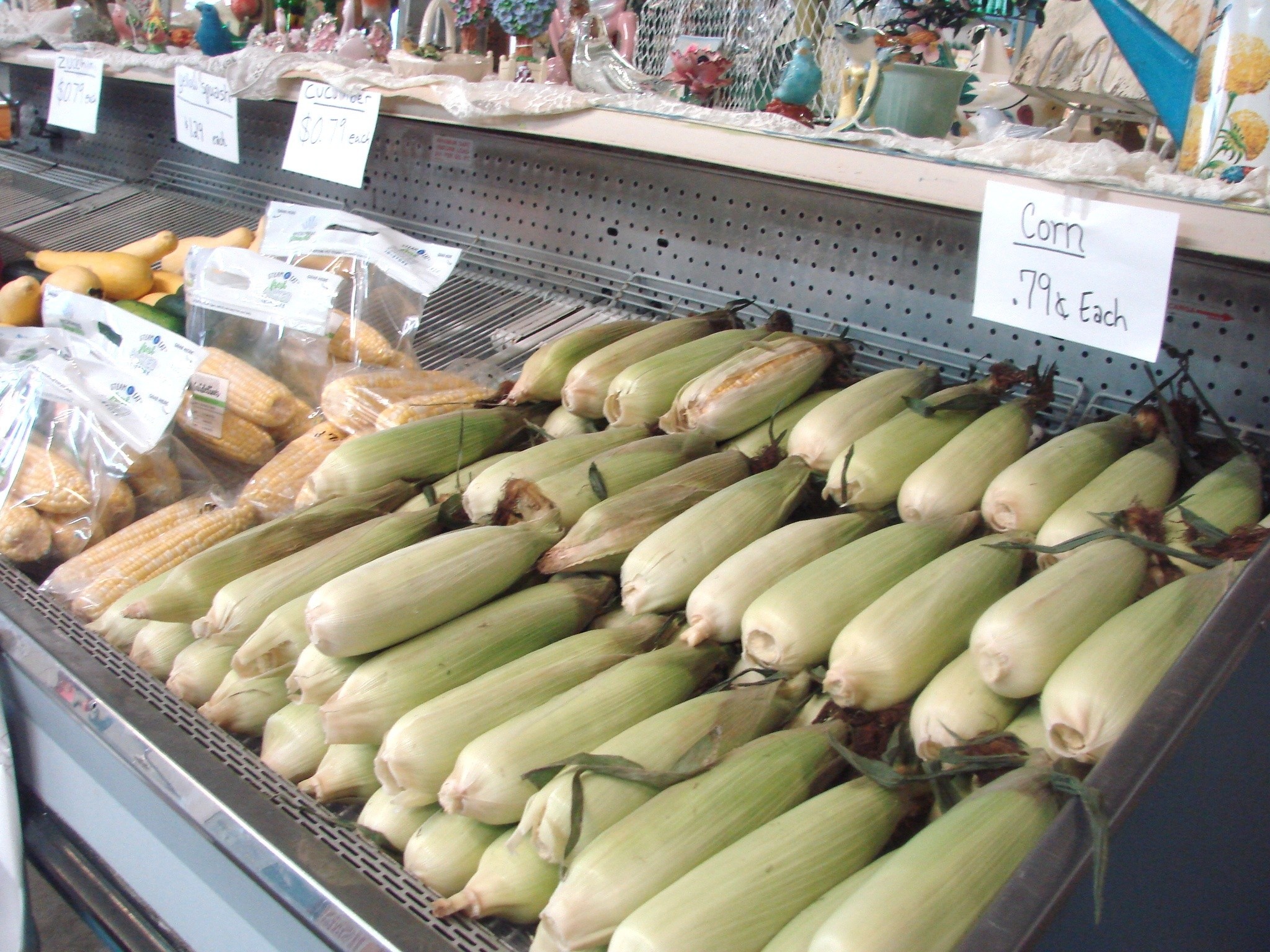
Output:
[386,50,490,83]
[870,61,973,140]
[951,73,1066,137]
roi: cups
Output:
[0,100,20,145]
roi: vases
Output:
[870,61,973,141]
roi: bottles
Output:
[973,25,1013,73]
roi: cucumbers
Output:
[110,284,187,339]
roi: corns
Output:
[0,297,505,622]
[84,311,1270,952]
